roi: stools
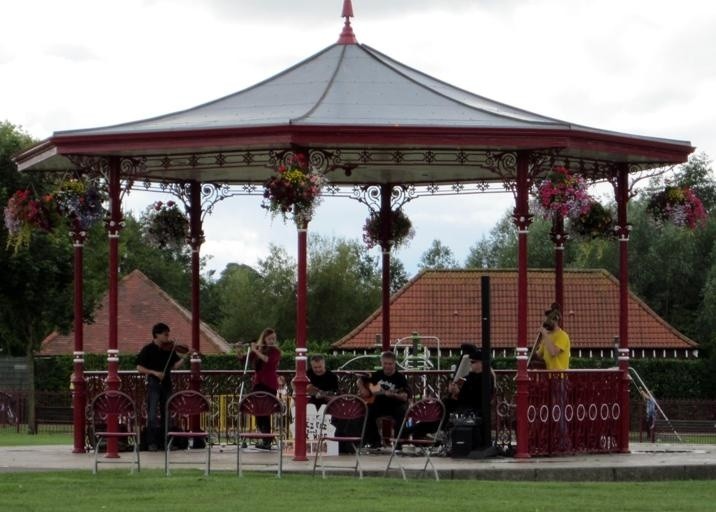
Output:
[376,416,395,445]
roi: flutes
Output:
[224,342,278,347]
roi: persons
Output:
[135,322,195,427]
[306,355,338,410]
[533,310,571,371]
[236,328,282,449]
[357,351,412,455]
[440,351,495,432]
[640,386,656,442]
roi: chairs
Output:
[236,391,282,478]
[311,395,368,480]
[165,390,212,476]
[385,398,446,481]
[90,390,141,476]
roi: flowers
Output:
[261,152,329,223]
[147,200,189,251]
[4,184,127,247]
[362,210,411,250]
[647,183,709,233]
[536,164,615,244]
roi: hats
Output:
[469,349,481,359]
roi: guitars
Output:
[356,383,408,405]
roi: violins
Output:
[161,341,206,362]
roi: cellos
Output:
[513,302,560,394]
